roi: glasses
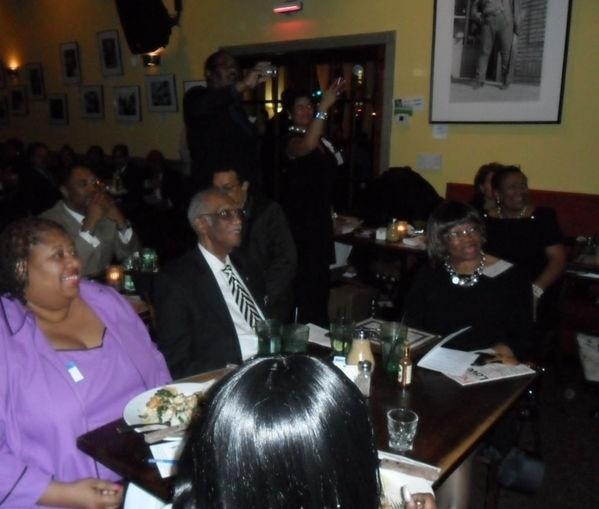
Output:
[198,208,247,221]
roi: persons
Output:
[2,138,184,277]
[0,217,173,509]
[166,52,347,373]
[469,163,566,330]
[165,353,438,509]
[471,0,519,90]
[406,200,529,359]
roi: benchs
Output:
[446,183,599,352]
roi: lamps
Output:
[4,57,22,84]
[116,0,183,54]
[139,52,162,66]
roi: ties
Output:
[222,264,264,335]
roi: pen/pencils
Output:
[148,459,180,464]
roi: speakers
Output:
[114,1,171,54]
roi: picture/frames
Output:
[112,85,143,121]
[24,63,45,101]
[9,85,27,114]
[59,42,81,84]
[429,0,571,125]
[45,93,66,123]
[79,85,103,119]
[143,72,178,112]
[96,28,123,77]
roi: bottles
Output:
[398,346,412,385]
[387,217,400,242]
[355,360,373,396]
[348,330,375,373]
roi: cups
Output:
[123,248,157,291]
[387,408,418,455]
[330,320,356,355]
[256,320,283,353]
[285,323,310,352]
[376,322,408,372]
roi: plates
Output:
[123,382,203,441]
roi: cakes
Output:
[141,388,201,426]
